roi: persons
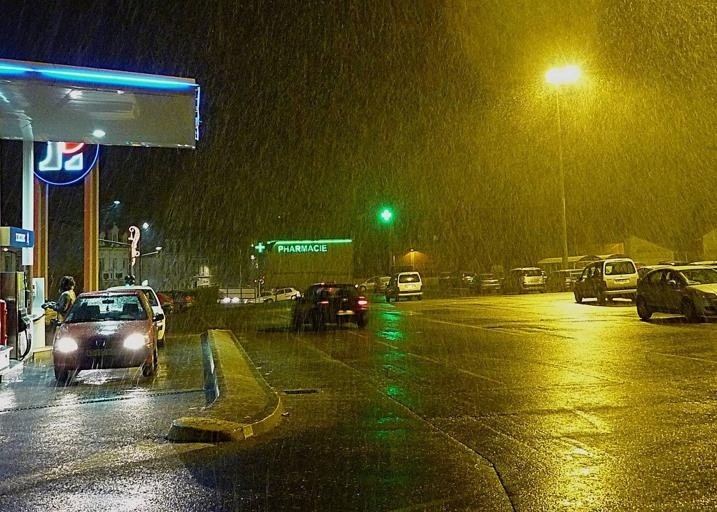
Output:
[43,276,76,329]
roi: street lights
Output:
[379,210,393,272]
[138,223,148,286]
[253,243,267,292]
[541,62,579,256]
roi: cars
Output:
[250,272,424,330]
[52,286,196,384]
[436,258,717,319]
